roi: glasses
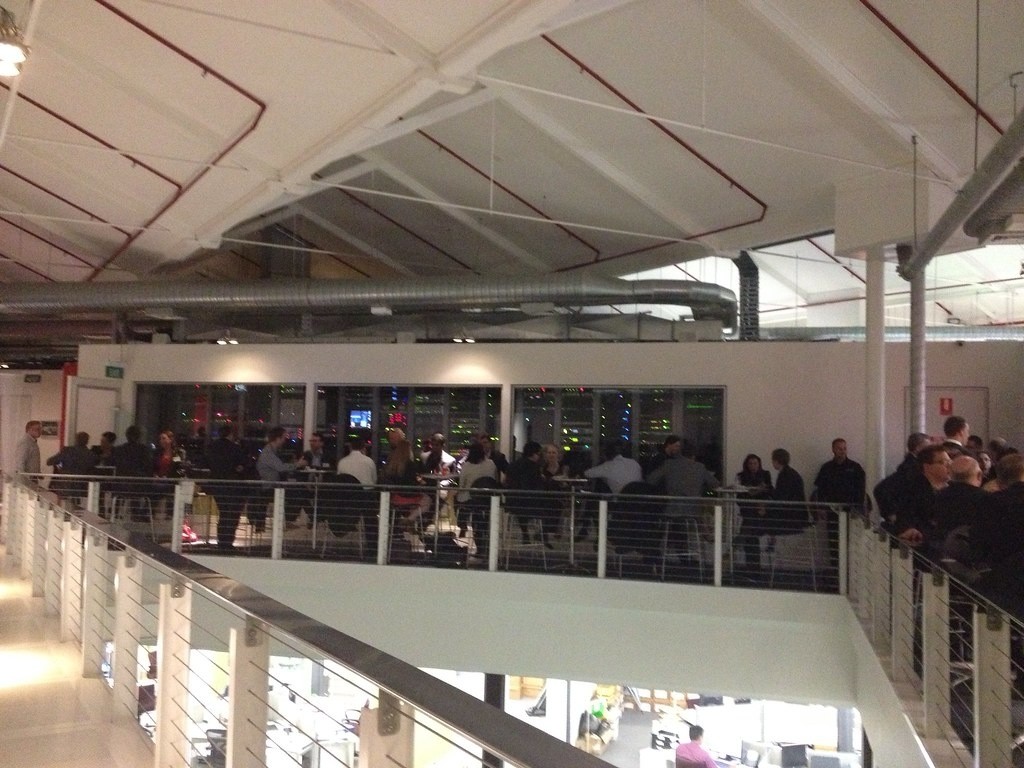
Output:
[930,459,951,466]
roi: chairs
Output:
[69,464,844,589]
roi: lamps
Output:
[0,5,32,78]
[217,329,240,345]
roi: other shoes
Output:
[534,534,553,549]
[573,529,588,544]
[521,535,530,544]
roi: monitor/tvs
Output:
[739,741,763,768]
[782,744,807,768]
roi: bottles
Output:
[452,461,456,475]
[442,461,447,475]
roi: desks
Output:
[420,472,459,558]
[554,476,589,565]
[714,484,753,579]
[297,469,334,551]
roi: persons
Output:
[870,417,1024,703]
[253,427,512,559]
[46,427,193,523]
[504,434,719,564]
[208,425,258,550]
[724,447,808,569]
[672,723,719,768]
[811,438,866,569]
[11,420,44,486]
